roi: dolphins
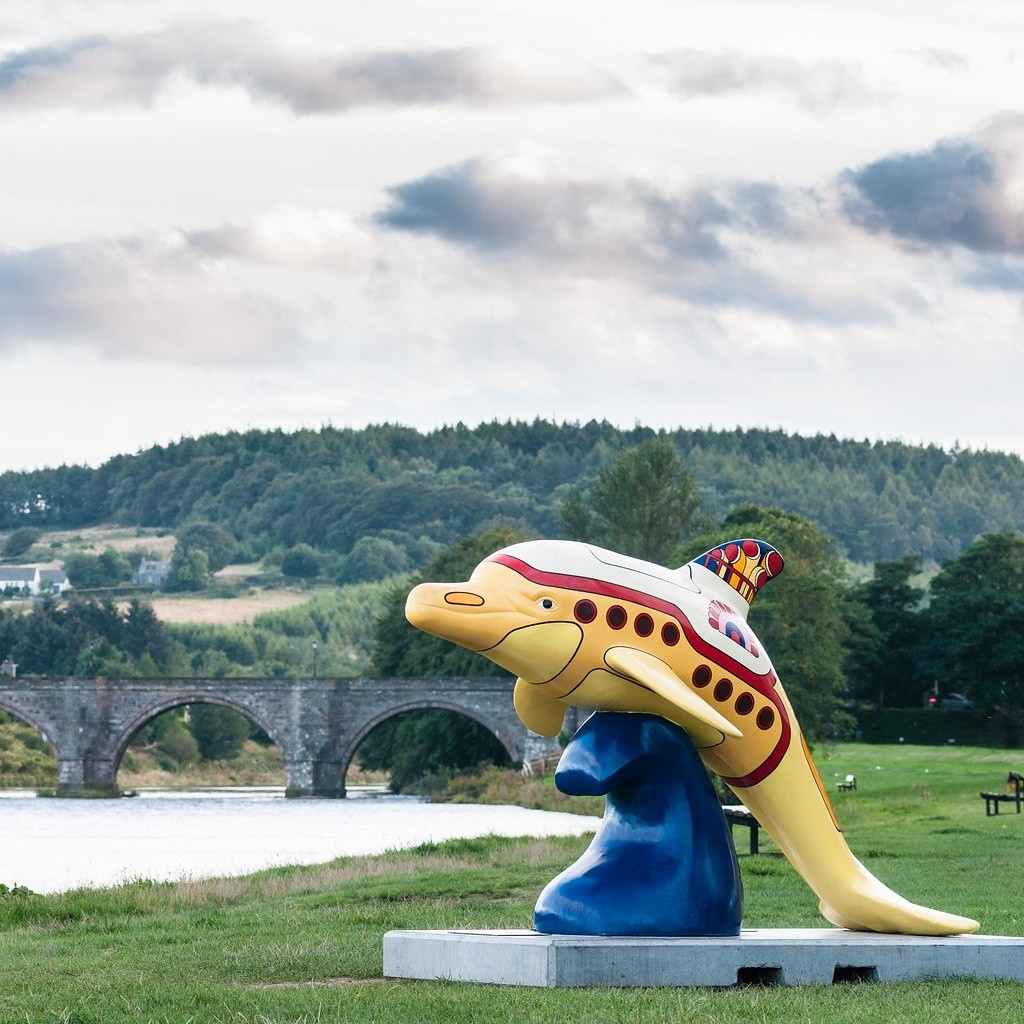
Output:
[405,537,983,938]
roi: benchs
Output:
[720,802,764,856]
[835,774,858,793]
[980,771,1024,816]
